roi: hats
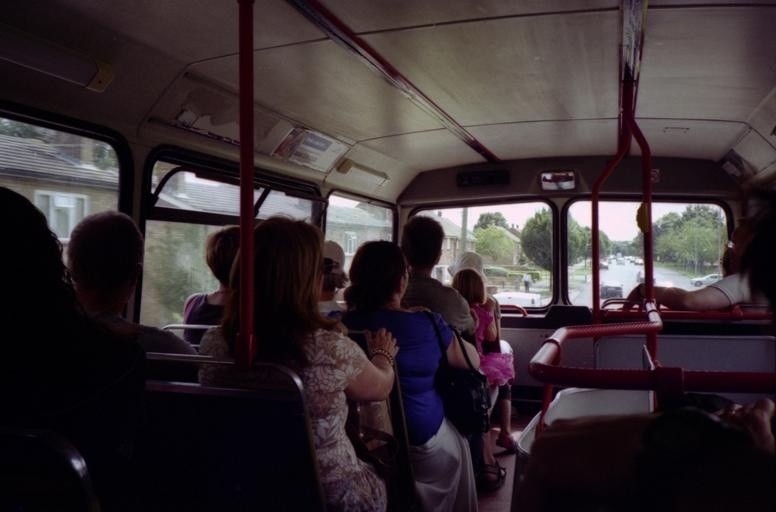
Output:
[447,252,490,285]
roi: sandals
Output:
[480,460,507,489]
[496,435,518,454]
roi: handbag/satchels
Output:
[436,364,491,438]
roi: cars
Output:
[617,258,625,265]
[630,257,636,263]
[600,260,608,269]
[691,273,722,287]
[635,269,656,282]
[635,259,644,265]
[601,280,623,297]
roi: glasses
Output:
[323,258,332,273]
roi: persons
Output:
[185,216,517,512]
[629,396,774,512]
[623,207,776,325]
[1,186,199,512]
[524,272,530,292]
